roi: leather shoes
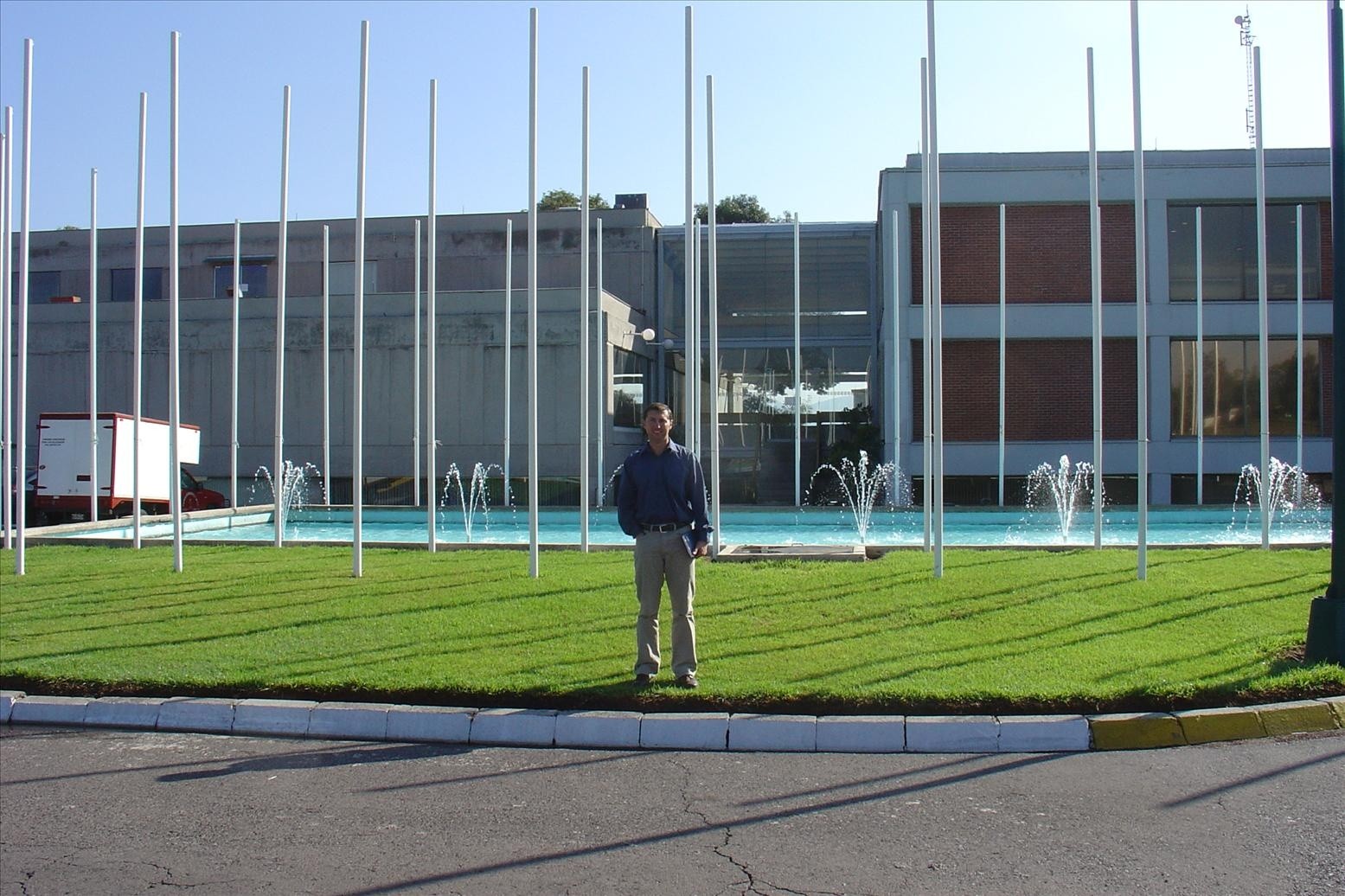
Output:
[632,673,654,686]
[680,674,697,688]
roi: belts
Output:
[639,521,690,534]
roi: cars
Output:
[1,471,37,525]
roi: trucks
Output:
[34,411,225,526]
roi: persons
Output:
[617,402,715,688]
